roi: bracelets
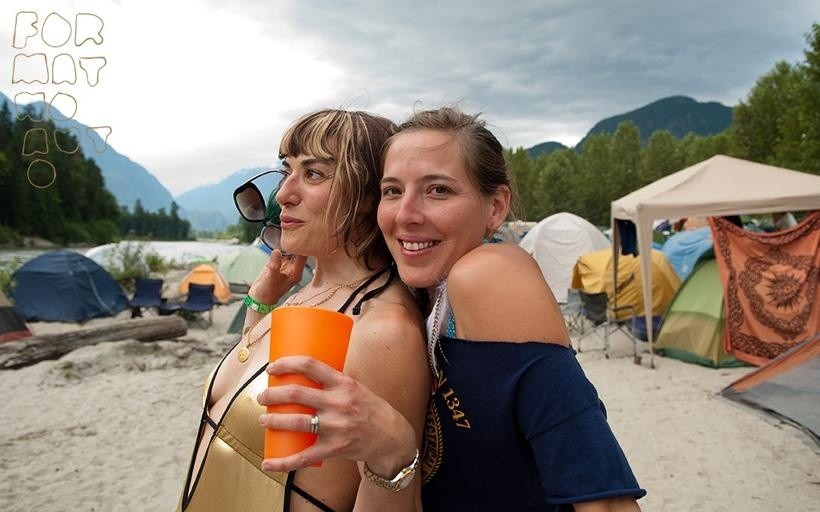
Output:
[242,291,281,315]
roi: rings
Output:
[310,413,320,435]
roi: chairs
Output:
[577,290,636,359]
[130,275,161,318]
[180,282,215,329]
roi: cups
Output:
[264,308,352,468]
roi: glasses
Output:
[232,169,293,256]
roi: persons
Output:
[175,106,435,511]
[374,107,648,511]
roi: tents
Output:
[225,238,315,298]
[715,332,819,447]
[4,249,131,325]
[175,261,233,306]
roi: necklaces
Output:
[238,268,379,364]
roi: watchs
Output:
[361,448,422,493]
